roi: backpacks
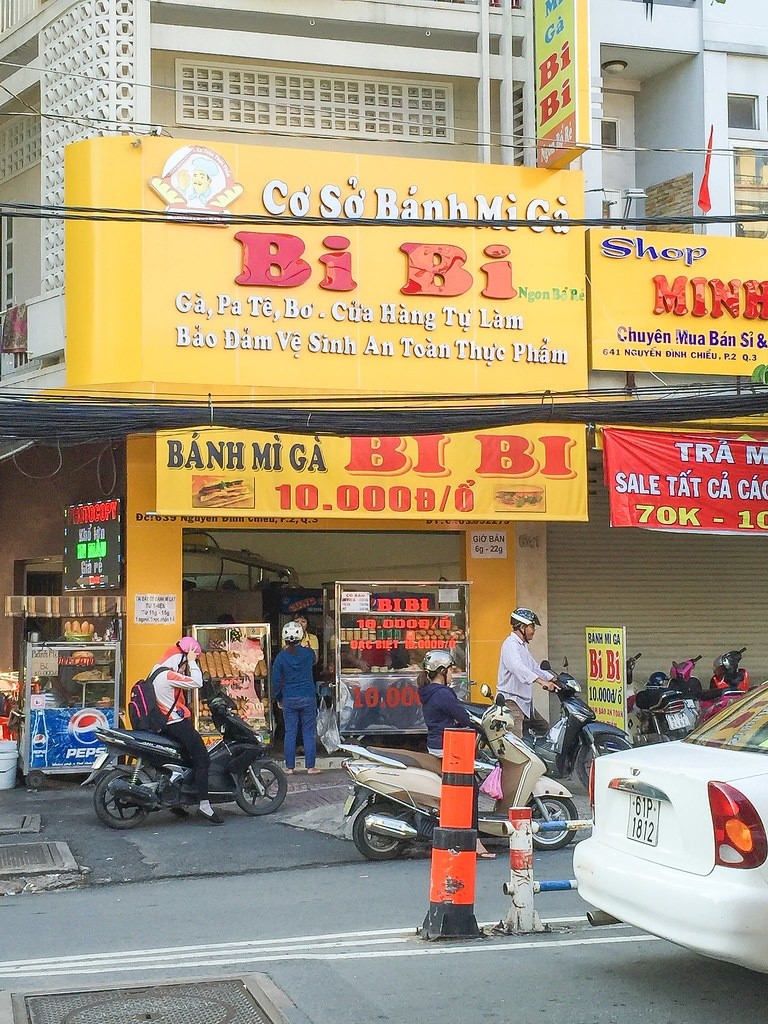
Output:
[128,667,182,734]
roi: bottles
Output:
[31,709,48,767]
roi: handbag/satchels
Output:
[547,716,568,754]
[316,696,342,755]
[478,761,504,800]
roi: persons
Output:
[282,607,319,755]
[144,643,224,823]
[347,613,465,748]
[417,649,497,859]
[271,622,323,774]
[494,607,558,739]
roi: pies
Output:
[197,479,253,508]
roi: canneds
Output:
[340,627,402,641]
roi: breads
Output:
[199,651,268,717]
[415,625,466,641]
[65,620,94,634]
[72,650,106,680]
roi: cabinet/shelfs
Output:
[323,579,472,672]
[188,624,272,731]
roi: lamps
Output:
[601,59,628,75]
[619,188,648,229]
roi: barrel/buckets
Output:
[0,751,19,790]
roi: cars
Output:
[572,679,768,978]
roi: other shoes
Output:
[307,768,323,774]
[196,806,225,825]
[171,808,188,818]
[281,768,294,775]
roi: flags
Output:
[698,135,713,213]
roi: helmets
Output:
[422,649,456,672]
[175,636,201,660]
[282,621,304,643]
[649,671,669,690]
[510,607,542,628]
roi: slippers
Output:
[476,852,498,860]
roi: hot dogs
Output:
[496,485,544,509]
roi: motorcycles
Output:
[644,655,746,727]
[337,682,579,861]
[626,652,691,749]
[709,647,761,693]
[78,670,289,829]
[458,655,635,792]
